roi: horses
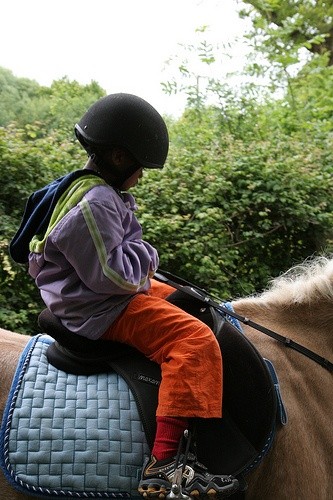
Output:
[1,258,333,500]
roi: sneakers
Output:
[138,449,240,499]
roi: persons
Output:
[8,92,243,499]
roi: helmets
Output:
[73,93,170,168]
